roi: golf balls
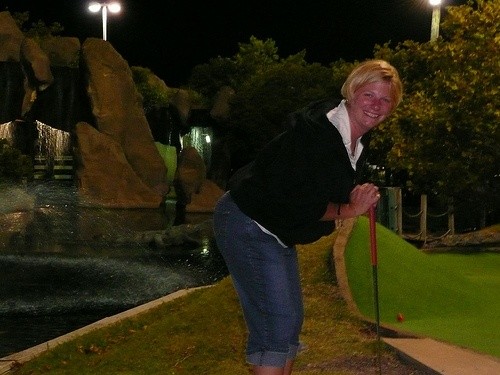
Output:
[397,313,403,322]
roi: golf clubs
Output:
[369,205,381,375]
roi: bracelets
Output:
[337,203,344,232]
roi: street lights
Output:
[88,1,120,42]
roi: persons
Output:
[214,59,402,374]
